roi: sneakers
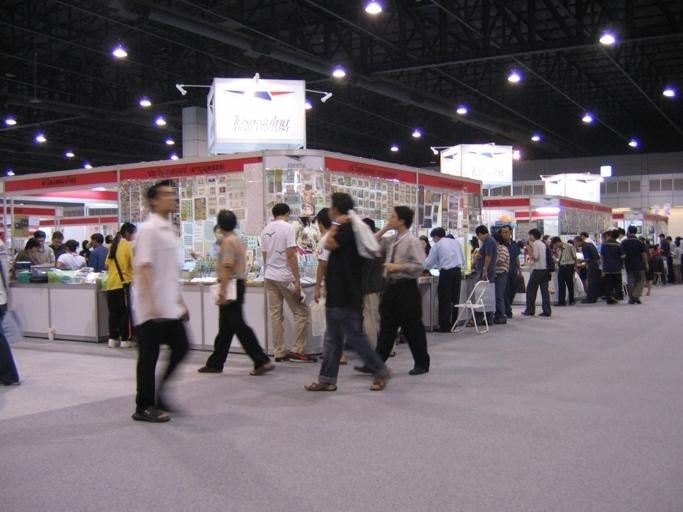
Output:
[0,379,19,385]
[494,319,506,324]
[504,312,512,318]
[108,338,120,347]
[521,312,534,315]
[435,327,450,332]
[275,353,288,362]
[289,352,318,363]
[120,341,136,347]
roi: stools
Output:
[655,272,662,288]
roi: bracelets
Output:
[332,220,342,226]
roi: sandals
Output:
[304,382,337,390]
[370,367,393,391]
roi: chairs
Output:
[451,280,489,334]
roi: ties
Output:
[384,246,396,288]
[558,250,563,266]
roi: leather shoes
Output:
[132,406,170,422]
[354,365,373,373]
[409,368,429,375]
[539,313,551,316]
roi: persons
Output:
[355,205,430,376]
[78,239,92,264]
[362,217,396,356]
[419,235,431,275]
[131,180,191,423]
[519,241,526,266]
[55,239,87,271]
[105,234,113,249]
[0,240,21,385]
[49,231,66,262]
[174,223,186,268]
[521,228,551,316]
[472,224,497,325]
[10,238,41,281]
[500,225,521,317]
[314,208,348,364]
[425,227,465,332]
[104,223,135,347]
[260,203,317,362]
[208,224,221,254]
[305,192,391,391]
[87,233,108,273]
[494,231,510,324]
[197,209,275,375]
[34,230,56,268]
[545,221,682,306]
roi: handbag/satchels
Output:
[310,298,326,336]
[121,283,129,312]
[348,209,382,259]
[2,310,24,344]
[512,276,525,292]
[475,283,496,312]
[546,246,555,272]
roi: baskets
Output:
[15,271,32,283]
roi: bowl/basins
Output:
[15,272,31,283]
[30,265,55,279]
[15,261,31,270]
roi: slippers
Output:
[198,366,223,372]
[249,363,275,375]
